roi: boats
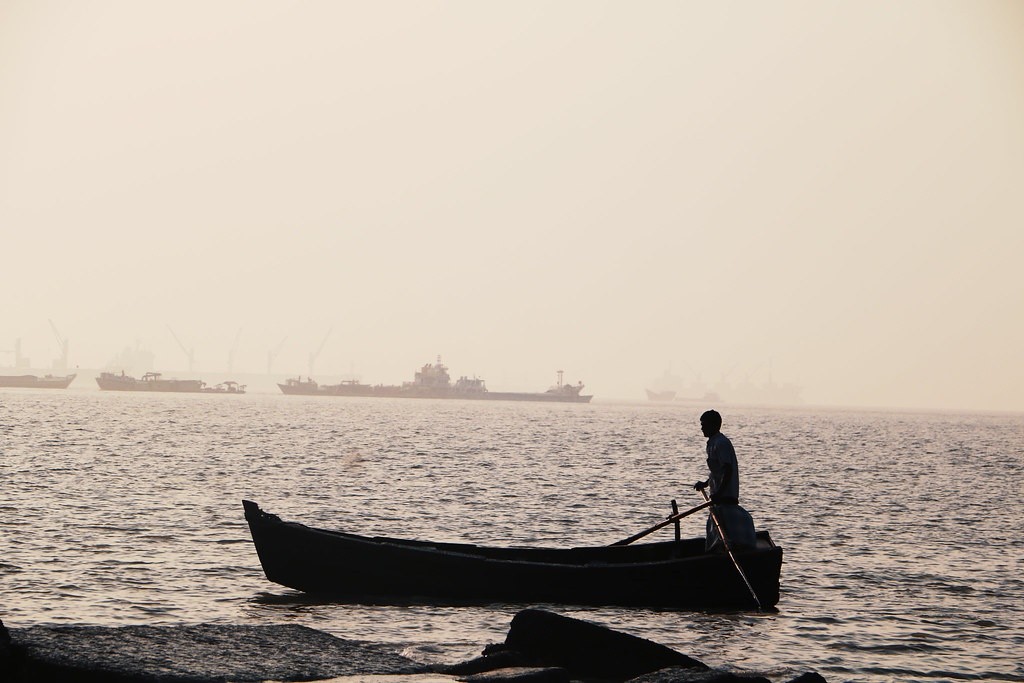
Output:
[277,376,318,395]
[242,489,783,614]
[95,371,206,392]
[438,370,593,403]
[338,379,377,397]
[0,373,77,388]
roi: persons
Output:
[693,409,739,554]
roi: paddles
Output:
[610,502,712,549]
[698,485,764,609]
